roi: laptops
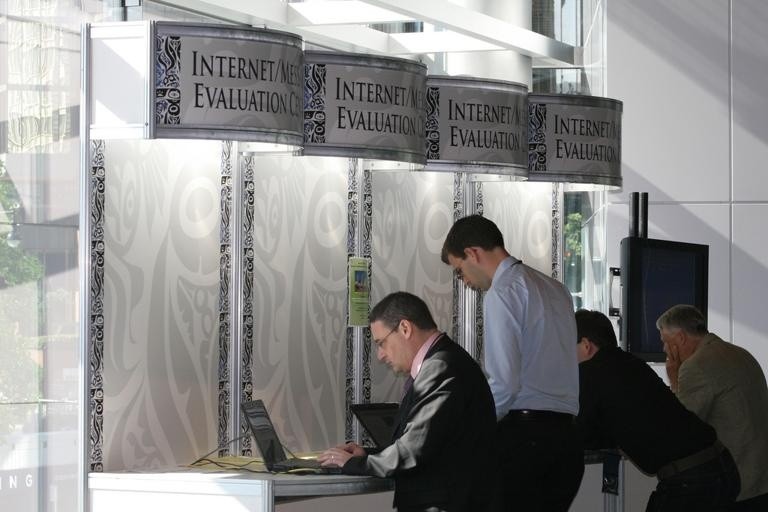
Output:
[241,400,344,474]
[350,403,400,448]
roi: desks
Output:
[88,446,629,511]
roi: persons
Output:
[574,308,742,511]
[315,291,507,511]
[440,214,587,511]
[654,302,768,510]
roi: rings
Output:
[331,454,334,461]
[666,356,670,361]
